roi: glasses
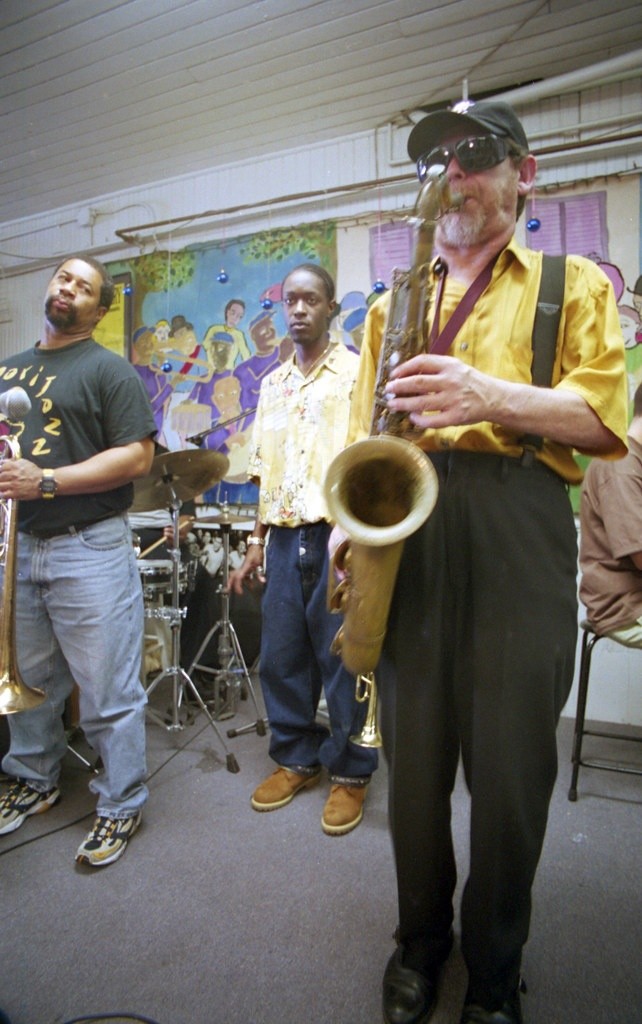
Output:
[415,133,512,185]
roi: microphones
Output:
[186,437,203,446]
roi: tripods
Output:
[94,482,267,774]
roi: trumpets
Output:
[349,672,384,750]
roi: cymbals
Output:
[187,512,255,525]
[127,448,230,513]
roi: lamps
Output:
[449,80,476,113]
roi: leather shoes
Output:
[379,927,455,1024]
[461,963,527,1024]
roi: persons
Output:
[187,529,262,581]
[578,380,642,651]
[131,433,226,706]
[228,264,378,837]
[0,254,157,868]
[343,100,630,1024]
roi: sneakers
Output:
[74,809,143,866]
[0,780,61,835]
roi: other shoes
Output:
[251,764,321,813]
[321,779,368,835]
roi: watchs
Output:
[38,469,58,499]
[245,535,266,549]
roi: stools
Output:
[567,620,642,800]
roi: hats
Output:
[407,100,528,223]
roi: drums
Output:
[137,558,190,596]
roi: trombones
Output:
[0,414,47,716]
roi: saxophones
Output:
[322,175,467,675]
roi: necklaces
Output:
[294,334,331,379]
